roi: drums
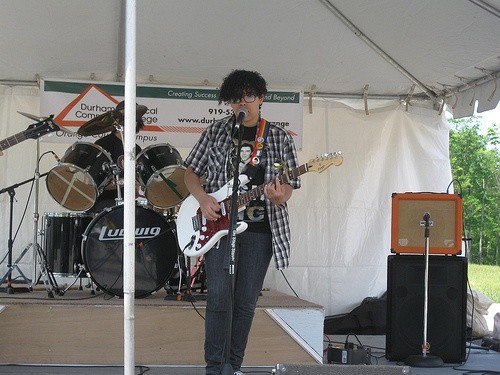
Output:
[44,141,116,213]
[42,212,96,279]
[80,198,179,300]
[135,142,191,211]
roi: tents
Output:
[0,0,500,301]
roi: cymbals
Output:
[16,110,76,137]
[75,104,148,136]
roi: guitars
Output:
[175,150,344,260]
[0,112,61,151]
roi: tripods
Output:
[0,138,60,298]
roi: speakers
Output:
[391,193,462,256]
[274,362,413,375]
[386,256,468,364]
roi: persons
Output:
[86,100,195,295]
[182,69,301,375]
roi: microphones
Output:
[234,107,249,130]
[423,211,430,220]
[52,151,60,162]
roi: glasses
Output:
[230,94,256,104]
